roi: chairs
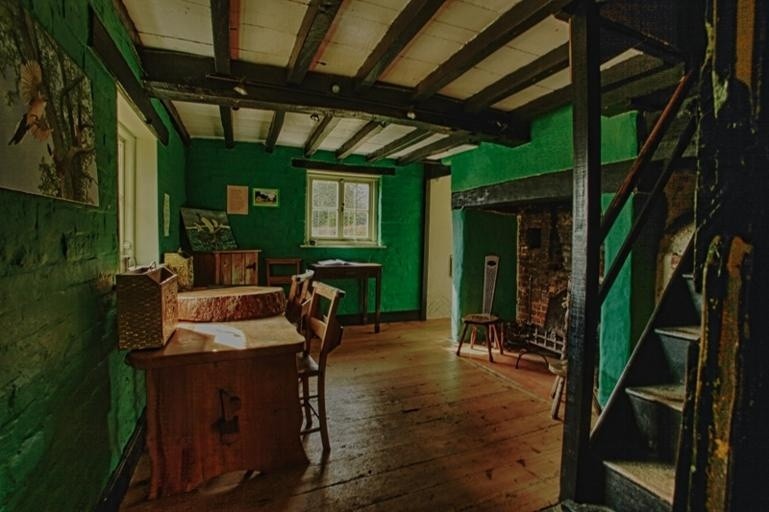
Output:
[297,281,345,449]
[285,269,315,323]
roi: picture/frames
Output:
[180,206,240,252]
[252,188,280,208]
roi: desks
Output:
[311,262,384,333]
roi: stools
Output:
[548,363,602,418]
[456,313,503,363]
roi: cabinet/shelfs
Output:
[194,249,264,286]
[125,317,306,501]
